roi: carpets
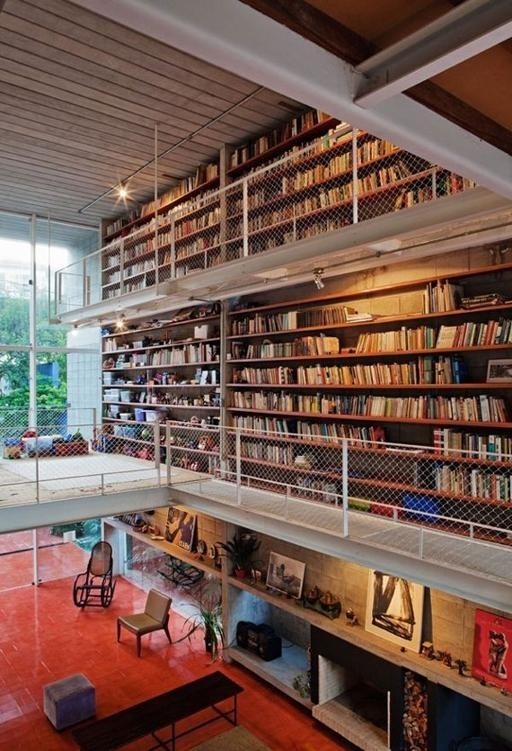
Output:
[187,724,272,751]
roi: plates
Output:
[196,540,216,559]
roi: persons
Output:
[267,554,283,587]
[167,510,193,550]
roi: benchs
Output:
[73,671,243,751]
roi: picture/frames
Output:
[364,569,425,654]
[162,507,197,553]
[265,552,308,600]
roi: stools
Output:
[43,674,95,732]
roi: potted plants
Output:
[175,582,225,668]
[213,526,262,585]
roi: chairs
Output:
[73,542,117,609]
[117,590,173,658]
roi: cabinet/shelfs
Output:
[99,239,512,548]
[101,107,512,301]
